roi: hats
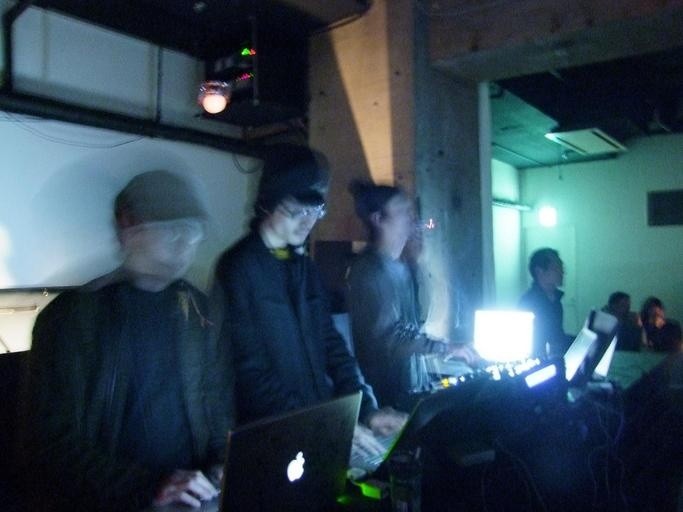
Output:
[114,171,206,228]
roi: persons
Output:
[343,176,481,410]
[210,141,443,461]
[23,169,242,510]
[637,296,682,354]
[601,291,641,352]
[514,249,576,362]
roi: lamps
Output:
[198,78,234,115]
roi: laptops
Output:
[564,326,599,382]
[351,377,491,474]
[218,391,364,512]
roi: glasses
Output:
[281,202,326,218]
